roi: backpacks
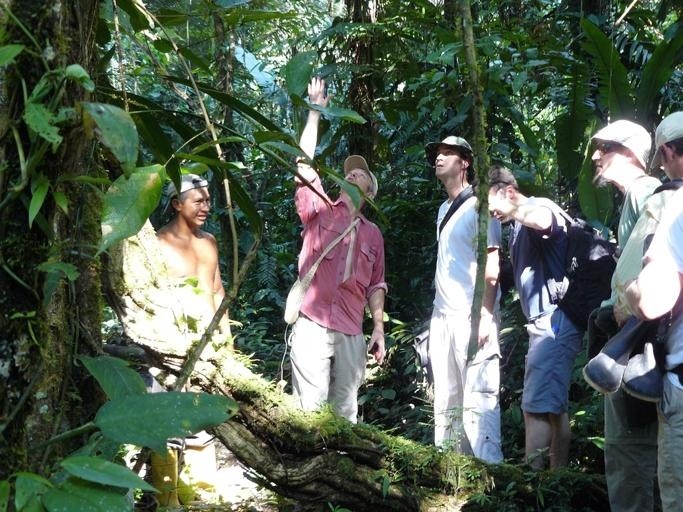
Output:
[284,216,364,325]
[527,197,617,331]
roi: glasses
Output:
[591,140,622,155]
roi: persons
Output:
[155,174,233,482]
[425,135,504,464]
[591,119,668,511]
[476,166,622,470]
[284,76,388,423]
[614,110,683,512]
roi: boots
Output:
[621,316,665,403]
[581,314,647,396]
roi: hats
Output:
[343,155,378,198]
[424,135,474,168]
[590,119,652,170]
[650,111,683,170]
[161,173,209,216]
